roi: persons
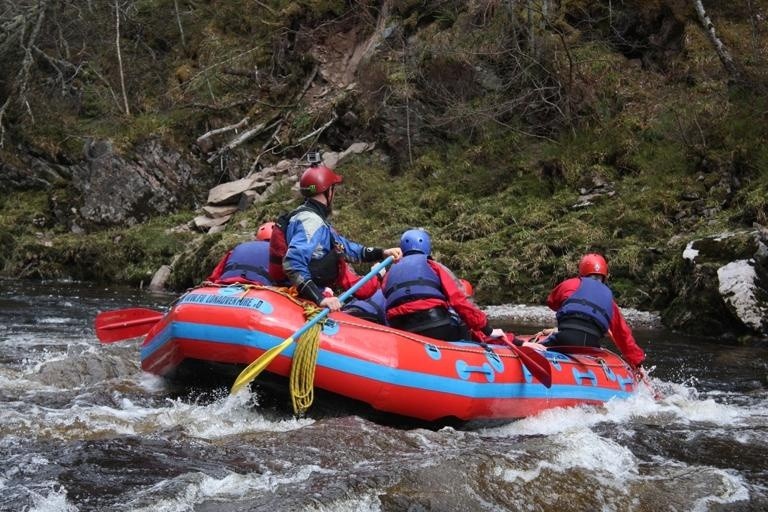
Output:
[268,165,403,313]
[381,229,511,343]
[205,220,277,287]
[535,251,651,387]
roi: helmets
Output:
[300,167,342,197]
[580,254,607,277]
[257,221,275,240]
[400,229,431,255]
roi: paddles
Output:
[95,309,166,342]
[499,336,553,389]
[231,255,396,394]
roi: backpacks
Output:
[269,206,326,284]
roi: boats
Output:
[139,280,637,428]
